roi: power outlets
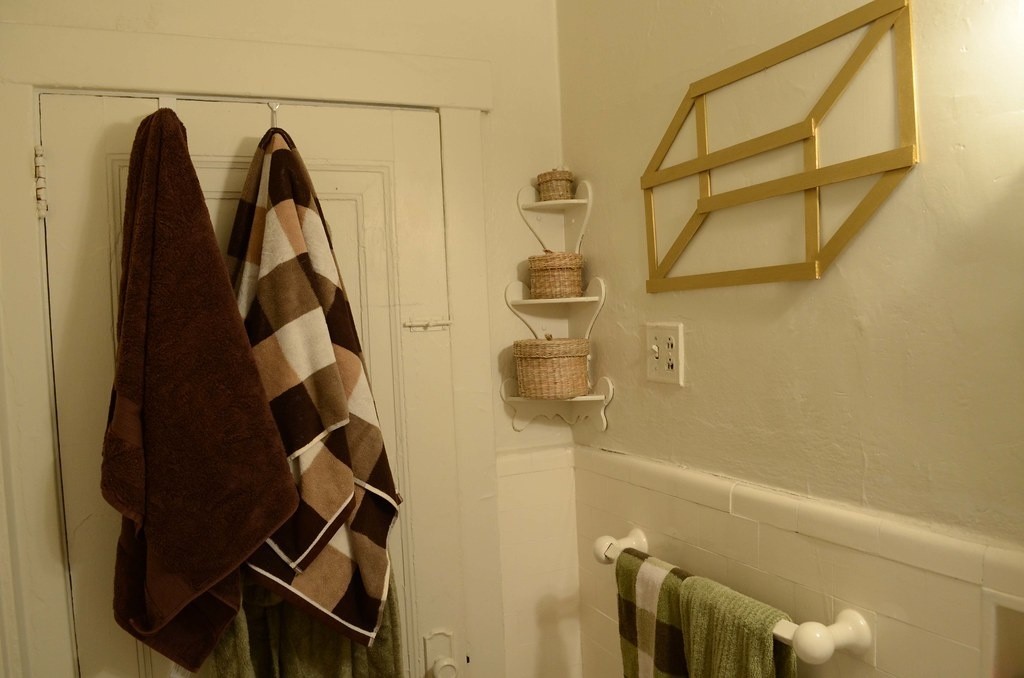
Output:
[646,322,684,386]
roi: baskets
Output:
[514,334,588,397]
[527,249,583,297]
[538,170,572,201]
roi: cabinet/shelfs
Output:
[500,180,613,432]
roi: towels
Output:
[96,106,303,676]
[611,545,800,678]
[167,126,410,678]
